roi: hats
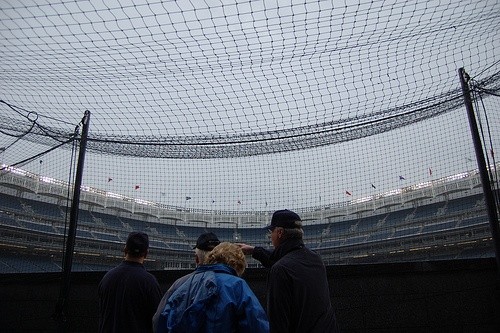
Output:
[265,209,304,230]
[193,232,220,251]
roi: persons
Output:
[157,242,269,333]
[152,232,222,332]
[97,232,163,333]
[233,210,341,333]
